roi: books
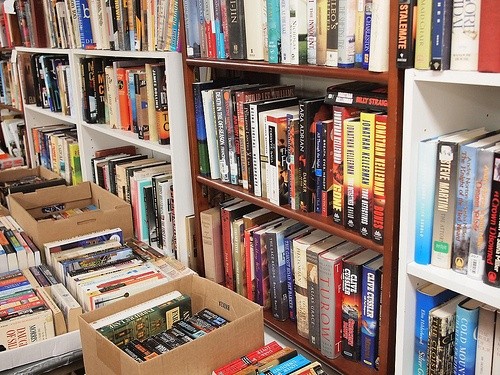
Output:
[0,1,500,375]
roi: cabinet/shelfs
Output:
[73,49,195,269]
[178,0,403,375]
[0,46,85,271]
[393,67,500,374]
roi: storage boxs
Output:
[6,179,134,242]
[79,275,264,374]
[0,165,65,208]
